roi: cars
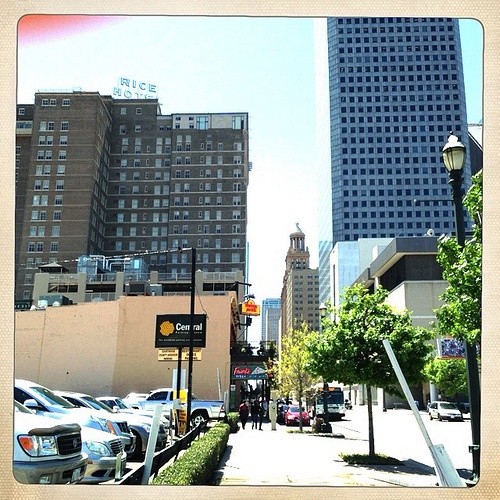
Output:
[13,378,226,485]
[428,401,462,422]
[284,406,311,427]
[277,401,294,425]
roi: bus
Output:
[306,382,346,421]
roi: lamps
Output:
[239,317,254,327]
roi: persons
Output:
[238,401,249,429]
[414,399,419,408]
[251,400,259,429]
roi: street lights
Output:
[440,126,480,487]
